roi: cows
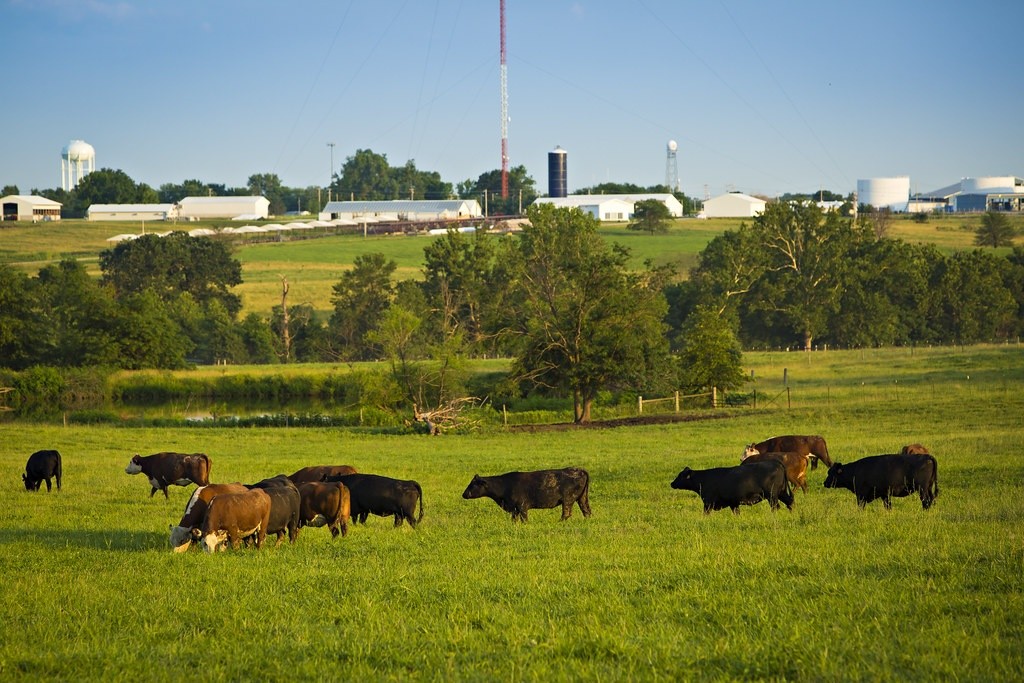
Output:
[21,450,62,492]
[463,468,592,524]
[125,451,424,555]
[672,436,938,514]
